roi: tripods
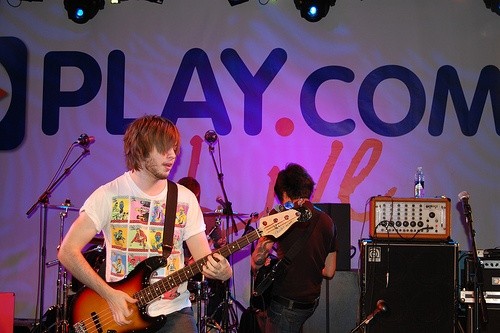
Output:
[188,150,276,333]
[27,146,76,333]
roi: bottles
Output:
[414,167,425,197]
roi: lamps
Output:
[63,0,105,24]
[293,0,336,23]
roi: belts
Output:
[272,294,319,310]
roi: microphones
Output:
[457,191,470,216]
[205,130,217,144]
[297,198,322,213]
[76,136,95,144]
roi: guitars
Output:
[71,204,312,333]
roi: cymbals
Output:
[203,210,248,216]
[42,204,79,211]
[87,238,103,245]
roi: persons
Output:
[57,114,233,333]
[254,162,339,333]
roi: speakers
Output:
[303,269,360,333]
[474,304,500,333]
[309,203,352,273]
[359,242,457,333]
